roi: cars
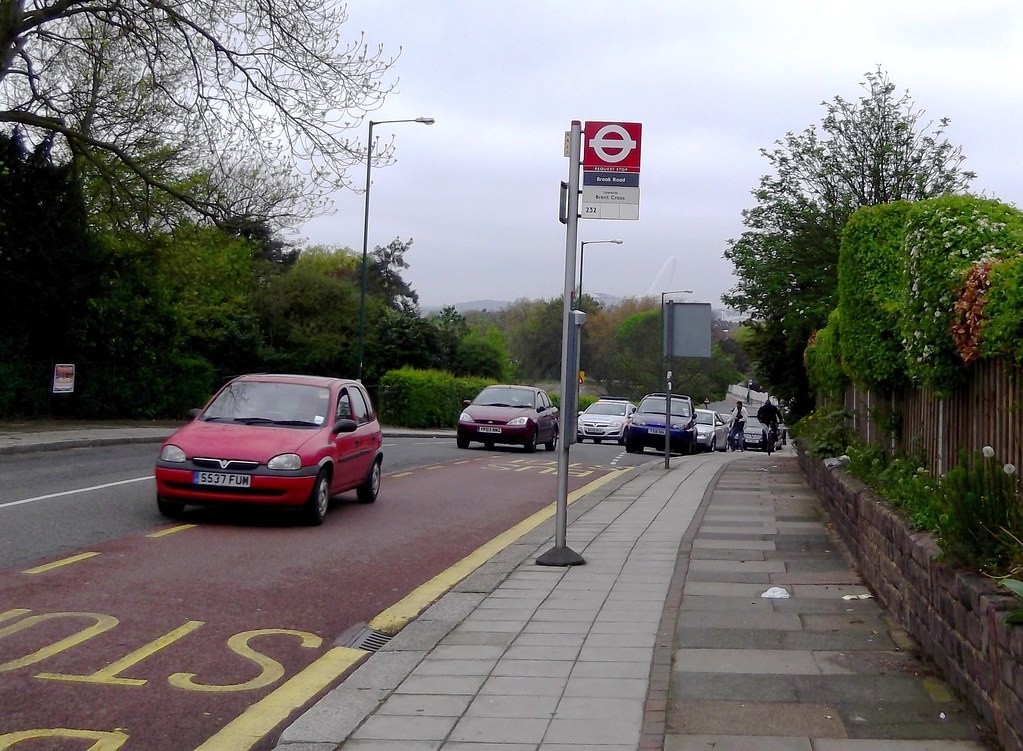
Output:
[154,374,384,526]
[457,384,559,454]
[683,408,730,453]
[733,415,771,451]
[626,393,698,456]
[720,414,732,426]
[577,400,638,445]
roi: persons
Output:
[727,401,748,451]
[298,390,322,418]
[757,400,782,452]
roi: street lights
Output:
[578,240,624,311]
[659,290,693,393]
[358,117,435,379]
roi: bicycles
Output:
[760,422,783,456]
